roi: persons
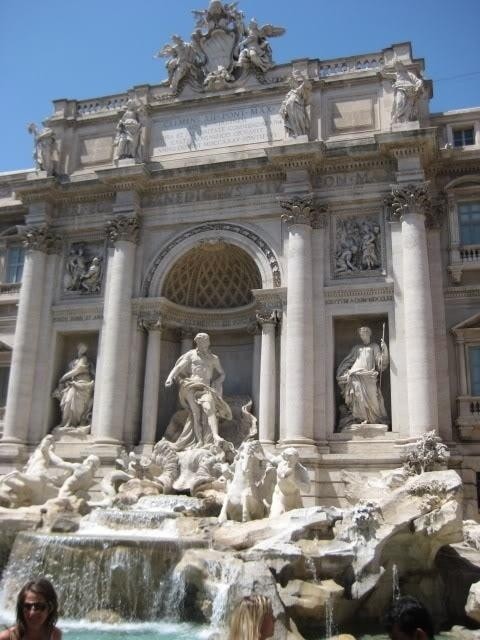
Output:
[51,343,94,427]
[165,333,232,448]
[113,100,142,161]
[66,248,103,294]
[280,70,310,136]
[28,118,55,177]
[47,445,101,513]
[158,0,266,89]
[228,594,276,640]
[336,327,389,424]
[384,596,434,640]
[334,225,380,274]
[381,60,423,121]
[0,579,61,640]
[262,449,311,517]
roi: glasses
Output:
[23,602,49,612]
